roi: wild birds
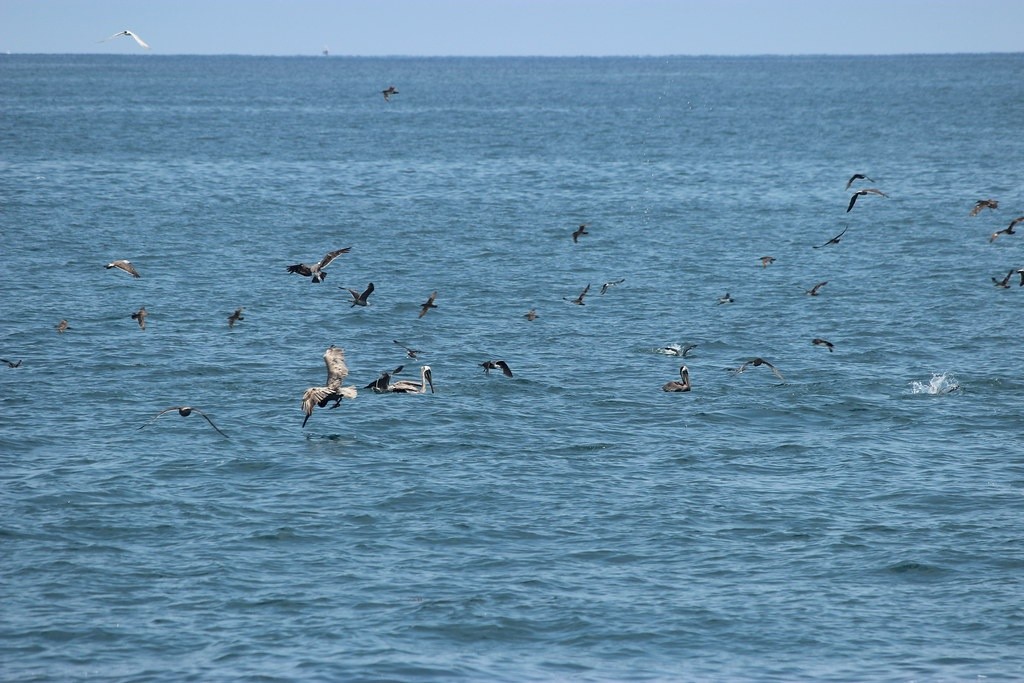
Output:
[302,345,359,426]
[57,319,67,334]
[104,260,141,279]
[131,307,148,331]
[0,358,24,369]
[217,307,244,328]
[418,291,439,319]
[564,283,590,305]
[136,406,230,440]
[285,246,352,285]
[479,359,513,377]
[369,363,435,395]
[600,278,626,295]
[393,339,429,359]
[523,309,539,322]
[571,224,587,244]
[105,30,149,50]
[662,169,1023,393]
[380,86,399,101]
[338,281,375,309]
